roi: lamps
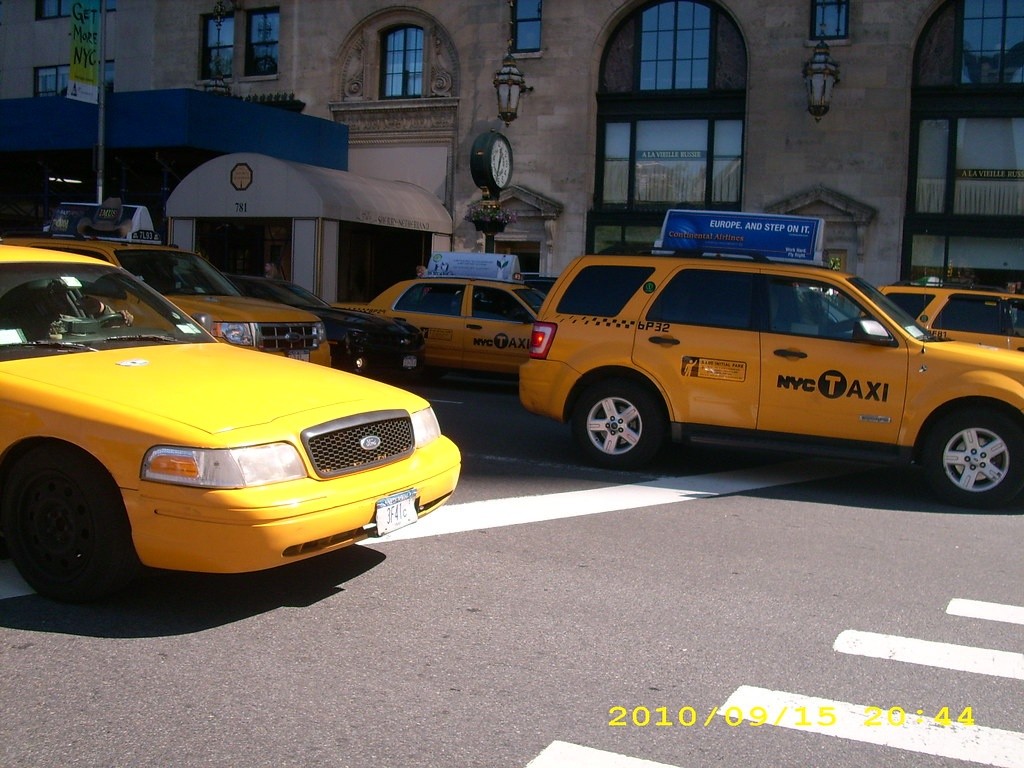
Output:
[255,12,276,71]
[203,1,232,93]
[492,0,533,128]
[801,0,840,124]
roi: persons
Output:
[138,252,175,296]
[191,246,209,272]
[265,261,285,280]
[784,290,873,337]
[169,244,179,249]
[18,273,135,342]
[416,265,431,298]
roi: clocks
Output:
[470,131,513,191]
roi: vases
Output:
[476,221,506,233]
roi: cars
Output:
[181,271,425,380]
[0,245,461,605]
[518,208,1024,511]
[0,198,332,367]
[852,281,1024,352]
[334,250,550,394]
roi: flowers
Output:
[462,206,517,223]
